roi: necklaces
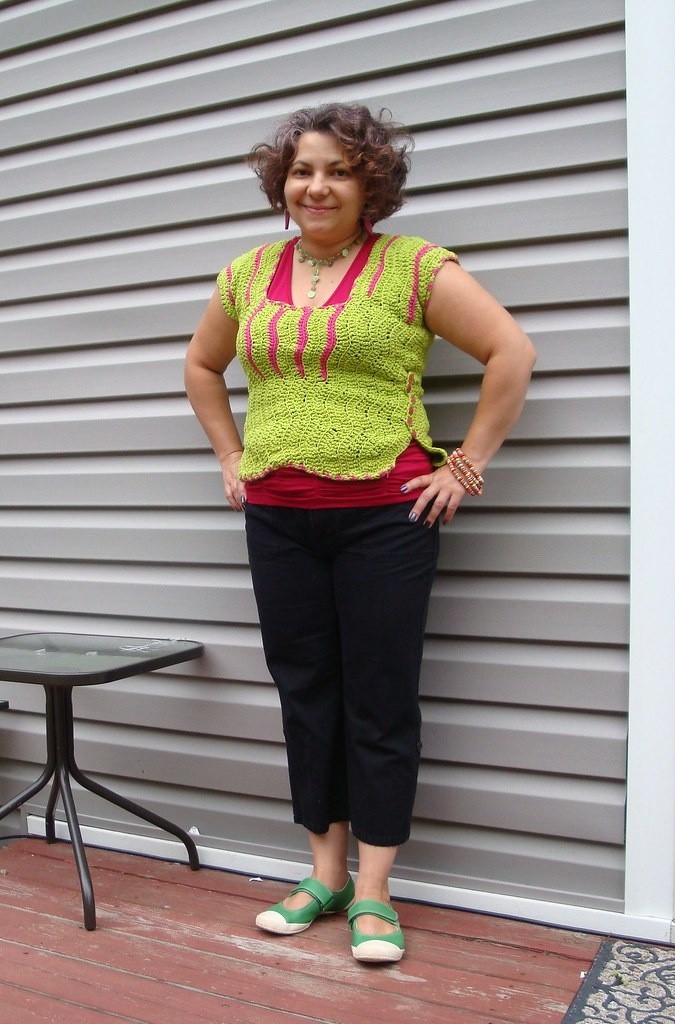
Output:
[296,238,361,298]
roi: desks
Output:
[0,632,205,930]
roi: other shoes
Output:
[254,872,356,935]
[347,899,404,962]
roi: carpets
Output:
[558,940,675,1024]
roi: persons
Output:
[184,105,536,963]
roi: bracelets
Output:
[447,448,483,495]
